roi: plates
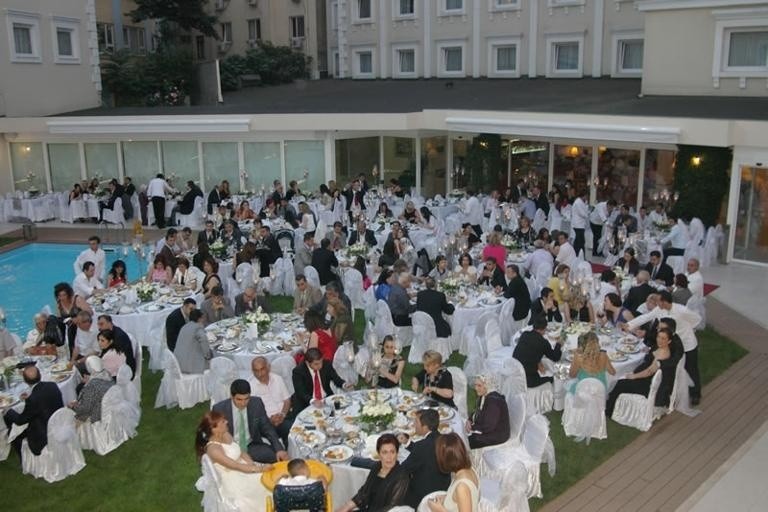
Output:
[400,395,425,406]
[616,336,639,345]
[296,429,327,449]
[213,343,237,354]
[0,395,21,408]
[172,289,192,297]
[321,443,354,462]
[167,296,184,304]
[140,304,165,313]
[607,355,629,363]
[615,345,641,355]
[297,409,329,424]
[47,373,70,384]
[477,297,502,307]
[48,362,67,373]
[326,395,351,411]
[426,407,455,421]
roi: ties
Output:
[314,370,322,400]
[239,411,247,451]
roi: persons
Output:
[1,149,706,512]
